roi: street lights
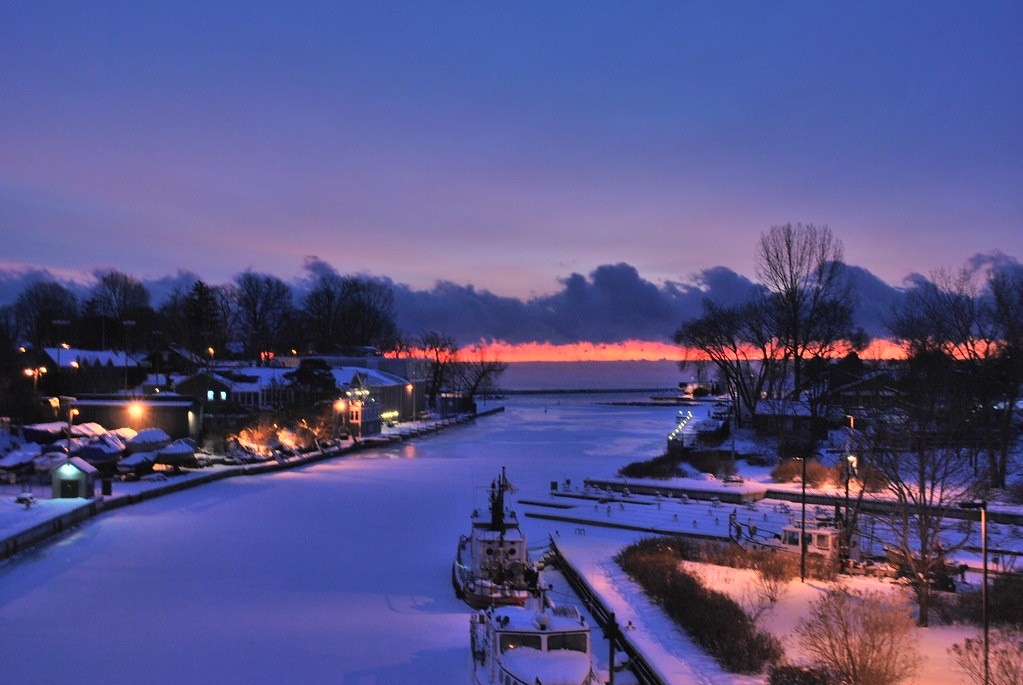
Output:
[24,366,47,400]
[956,498,988,685]
[791,454,807,582]
[67,409,79,460]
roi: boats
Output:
[469,529,612,685]
[1,421,196,475]
[452,465,541,611]
[725,508,970,581]
